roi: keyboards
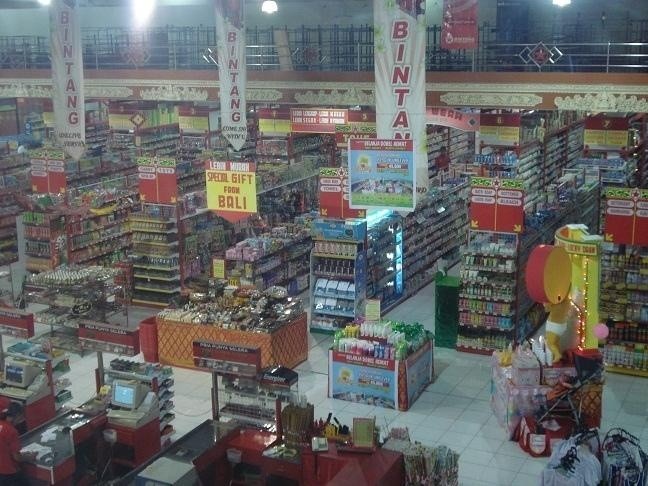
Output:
[4,387,33,397]
[107,410,145,419]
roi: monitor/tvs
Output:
[4,359,40,388]
[111,379,146,409]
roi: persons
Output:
[435,146,451,174]
